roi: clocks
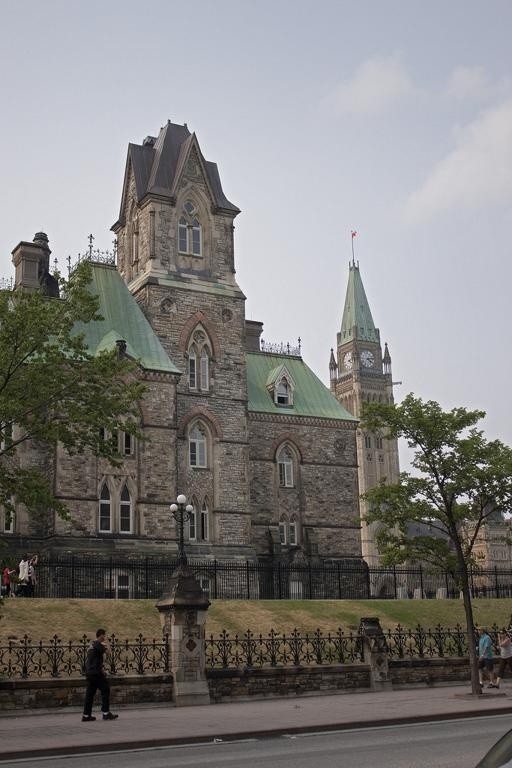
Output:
[360,349,375,368]
[343,352,354,370]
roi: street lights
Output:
[170,495,193,565]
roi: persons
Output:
[19,554,28,588]
[27,554,41,590]
[81,628,119,722]
[474,624,498,688]
[0,565,15,597]
[490,629,511,689]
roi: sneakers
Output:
[81,715,97,722]
[103,712,118,720]
[480,683,500,689]
